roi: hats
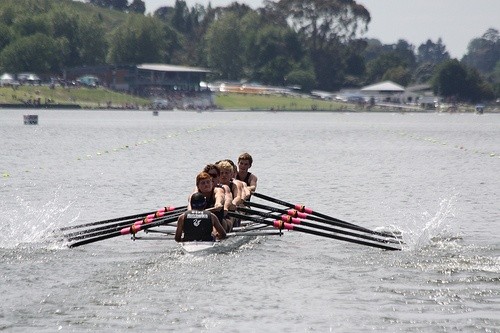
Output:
[190,192,206,206]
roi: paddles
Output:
[225,211,402,258]
[242,200,401,239]
[250,191,363,228]
[234,205,404,245]
[61,202,187,249]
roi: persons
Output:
[185,172,226,235]
[175,193,226,242]
[236,153,257,207]
[216,160,241,222]
[202,164,235,227]
[216,158,250,211]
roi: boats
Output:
[180,206,281,254]
